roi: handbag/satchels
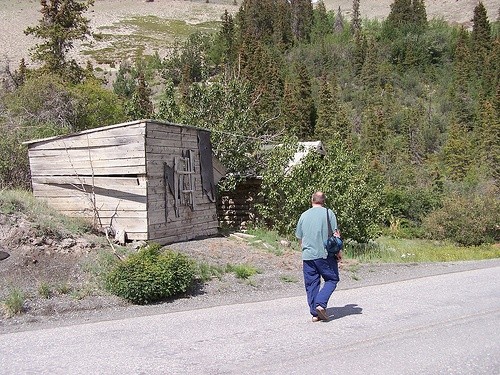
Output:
[326,206,343,253]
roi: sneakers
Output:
[311,306,329,322]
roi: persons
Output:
[295,192,343,322]
